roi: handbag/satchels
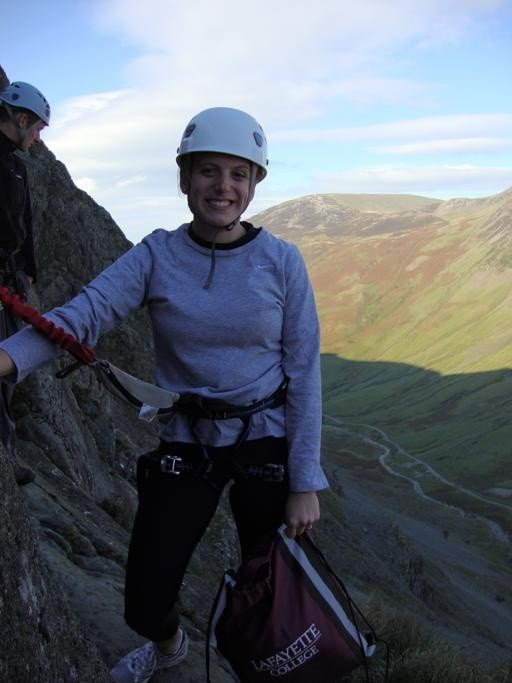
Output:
[206,526,390,682]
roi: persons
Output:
[1,105,330,680]
[1,80,51,486]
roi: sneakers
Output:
[108,630,189,683]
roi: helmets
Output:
[0,82,50,125]
[176,107,268,184]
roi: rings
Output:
[305,523,314,530]
